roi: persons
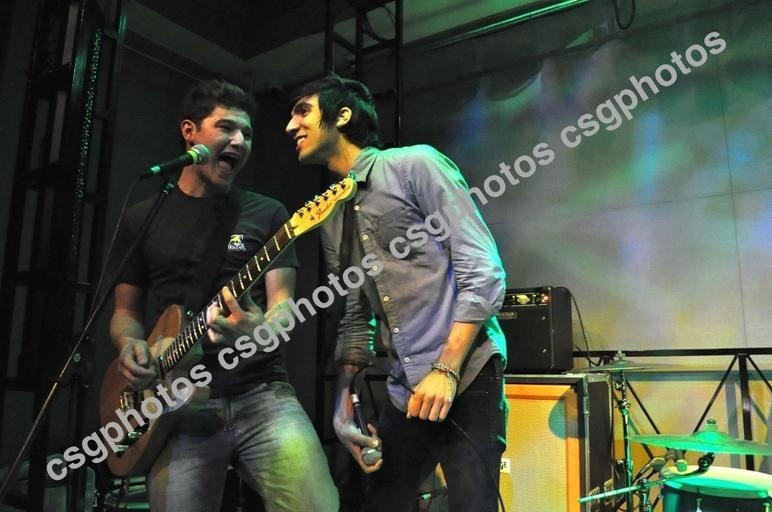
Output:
[110,74,509,512]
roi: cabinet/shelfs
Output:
[410,382,580,512]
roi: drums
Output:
[102,485,149,510]
[662,464,772,512]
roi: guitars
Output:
[98,172,358,478]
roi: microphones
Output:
[349,388,383,465]
[139,144,210,180]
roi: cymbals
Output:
[570,359,685,373]
[626,429,770,458]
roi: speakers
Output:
[496,286,574,374]
